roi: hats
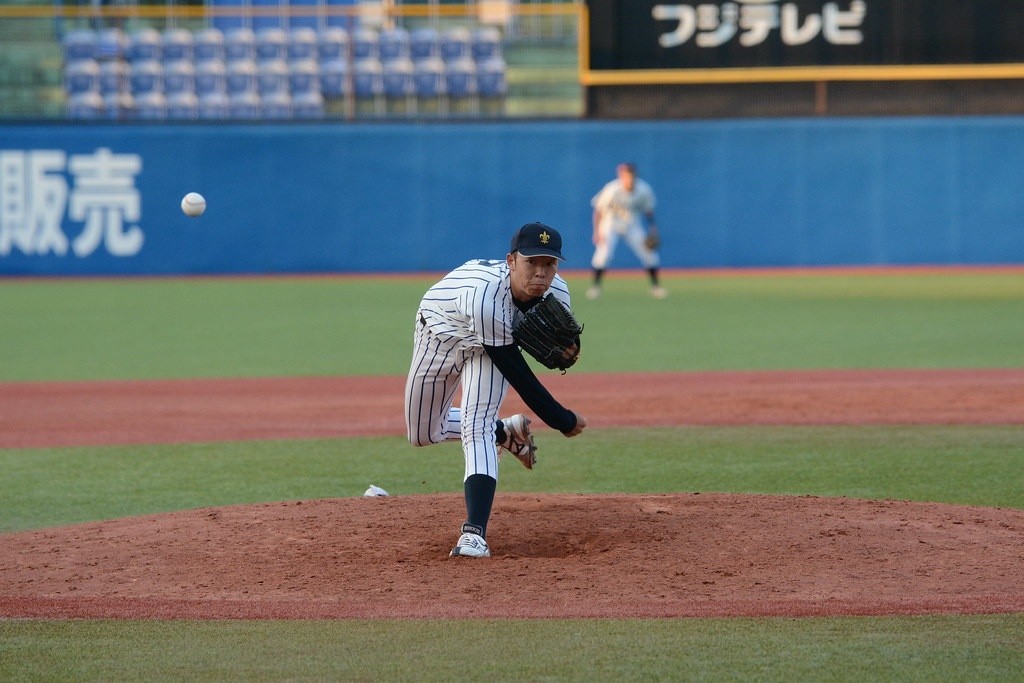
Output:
[511,222,566,262]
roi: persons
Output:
[587,163,665,298]
[405,221,588,558]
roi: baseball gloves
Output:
[645,233,659,249]
[511,292,587,376]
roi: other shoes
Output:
[586,286,601,299]
[652,287,666,298]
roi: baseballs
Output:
[181,191,207,218]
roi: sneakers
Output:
[449,521,490,558]
[495,414,537,471]
